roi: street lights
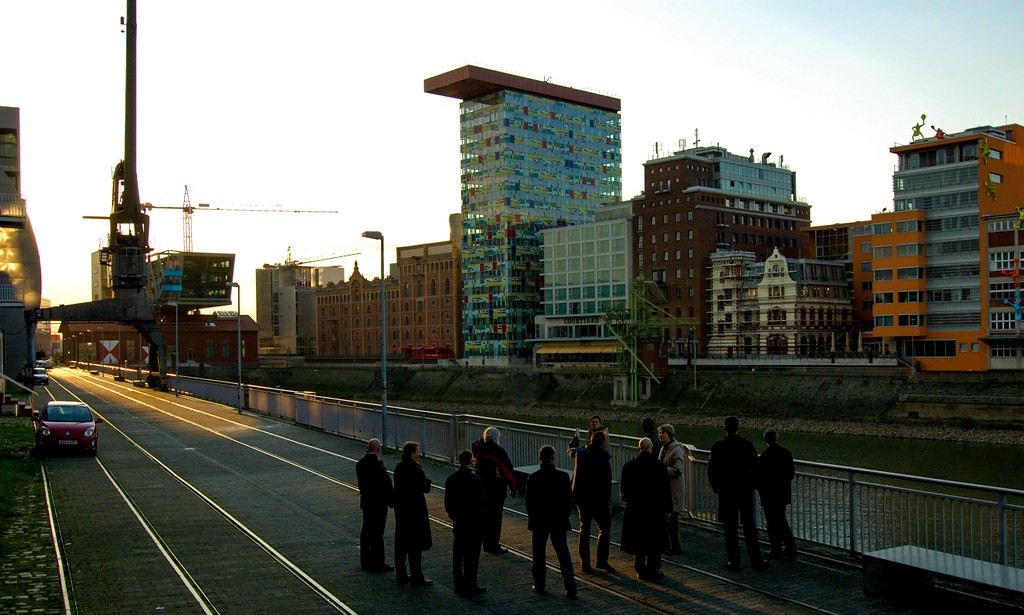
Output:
[167,301,179,399]
[78,333,85,343]
[360,231,388,457]
[87,329,94,364]
[224,281,243,414]
[71,334,78,365]
[96,326,105,377]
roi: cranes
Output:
[141,184,341,252]
[263,246,363,266]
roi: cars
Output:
[33,367,50,386]
[31,400,104,457]
[36,360,53,369]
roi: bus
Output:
[397,345,453,364]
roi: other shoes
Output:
[581,560,591,571]
[532,584,545,594]
[455,584,487,594]
[397,573,411,583]
[728,560,741,569]
[596,560,615,572]
[372,565,394,572]
[646,571,664,581]
[766,548,782,556]
[753,560,769,568]
[484,544,508,554]
[564,589,577,599]
[633,563,647,580]
[410,575,433,586]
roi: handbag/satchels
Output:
[664,516,681,555]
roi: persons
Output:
[657,424,685,554]
[356,439,394,572]
[472,427,517,554]
[525,445,577,598]
[621,437,672,579]
[643,418,662,459]
[756,428,798,562]
[393,442,433,587]
[445,451,488,598]
[587,415,613,513]
[707,417,768,569]
[567,430,616,575]
